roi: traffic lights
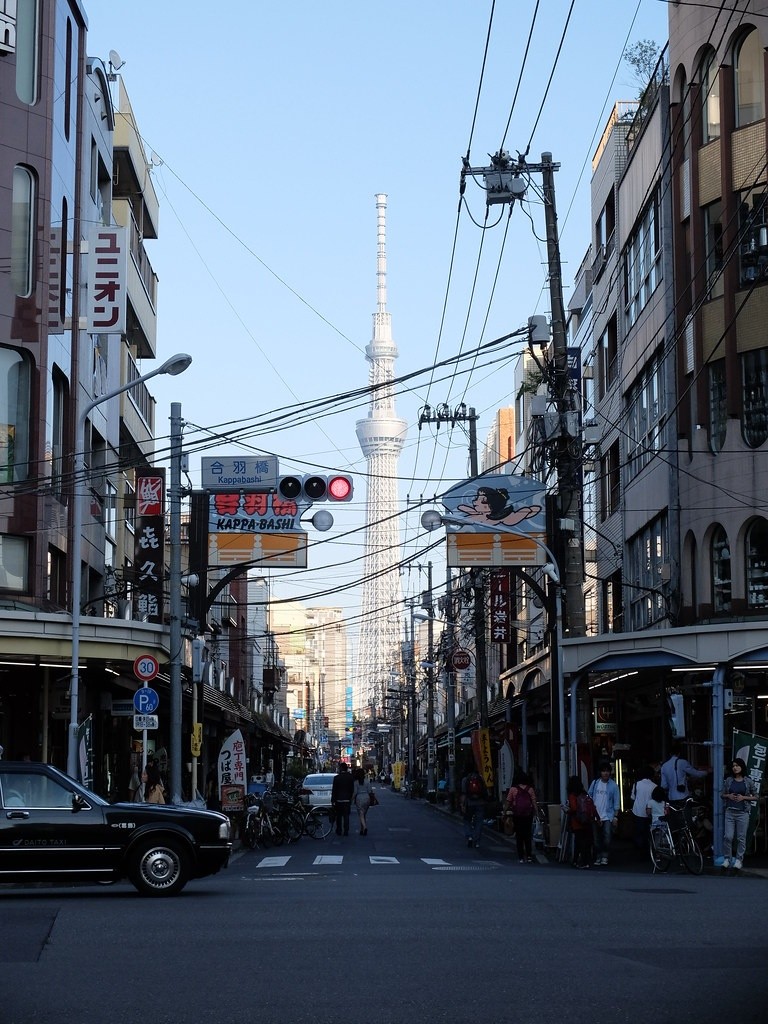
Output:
[278,475,355,502]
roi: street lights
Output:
[442,514,568,851]
[378,671,435,798]
[412,613,496,820]
[67,352,194,779]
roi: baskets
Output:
[263,801,273,813]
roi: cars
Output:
[1,763,232,896]
[300,774,338,812]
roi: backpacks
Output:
[467,777,482,798]
[512,784,533,816]
[577,791,596,824]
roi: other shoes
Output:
[468,837,473,848]
[520,858,535,864]
[360,828,367,835]
[475,844,480,848]
[594,857,609,866]
[721,859,732,869]
[576,864,590,868]
[732,859,743,870]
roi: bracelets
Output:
[614,816,618,821]
[502,814,505,816]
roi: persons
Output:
[128,754,275,810]
[719,757,760,869]
[460,764,486,848]
[329,762,448,836]
[561,742,712,868]
[501,772,540,864]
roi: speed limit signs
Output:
[133,655,160,681]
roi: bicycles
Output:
[381,776,384,787]
[649,798,704,875]
[239,791,333,851]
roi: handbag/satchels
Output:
[369,793,379,806]
[328,802,336,823]
